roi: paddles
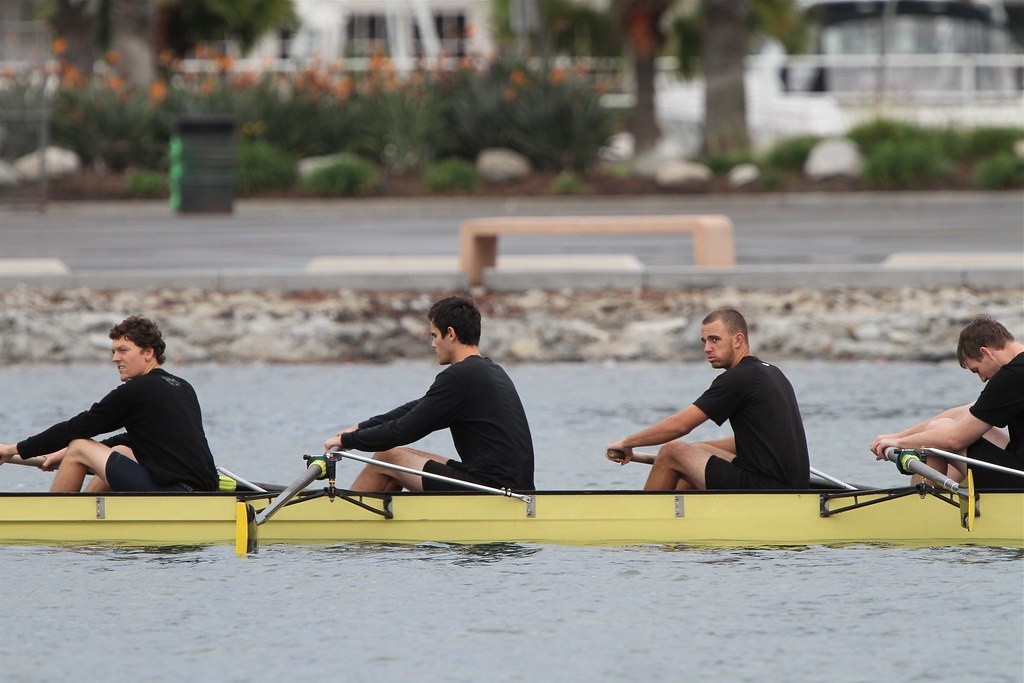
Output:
[6,454,289,491]
[234,445,341,553]
[608,449,880,491]
[883,446,982,534]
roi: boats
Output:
[0,482,1024,554]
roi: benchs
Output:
[458,215,736,276]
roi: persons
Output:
[871,312,1024,487]
[1,315,219,493]
[605,308,810,492]
[325,295,536,491]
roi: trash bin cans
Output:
[165,116,238,213]
